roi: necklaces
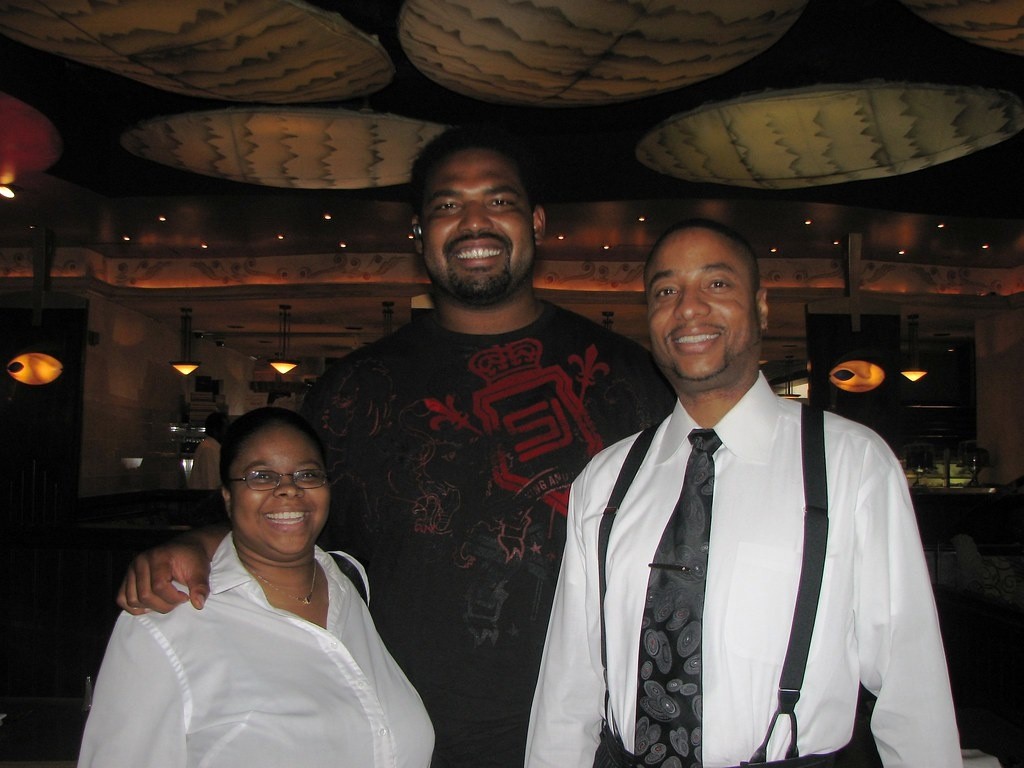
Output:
[254,558,318,604]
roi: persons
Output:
[78,407,435,768]
[525,218,962,768]
[188,412,230,490]
[117,127,679,767]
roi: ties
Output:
[632,426,721,768]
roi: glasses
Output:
[229,469,330,491]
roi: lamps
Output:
[169,307,199,376]
[269,305,297,374]
[900,314,927,382]
[9,226,62,385]
